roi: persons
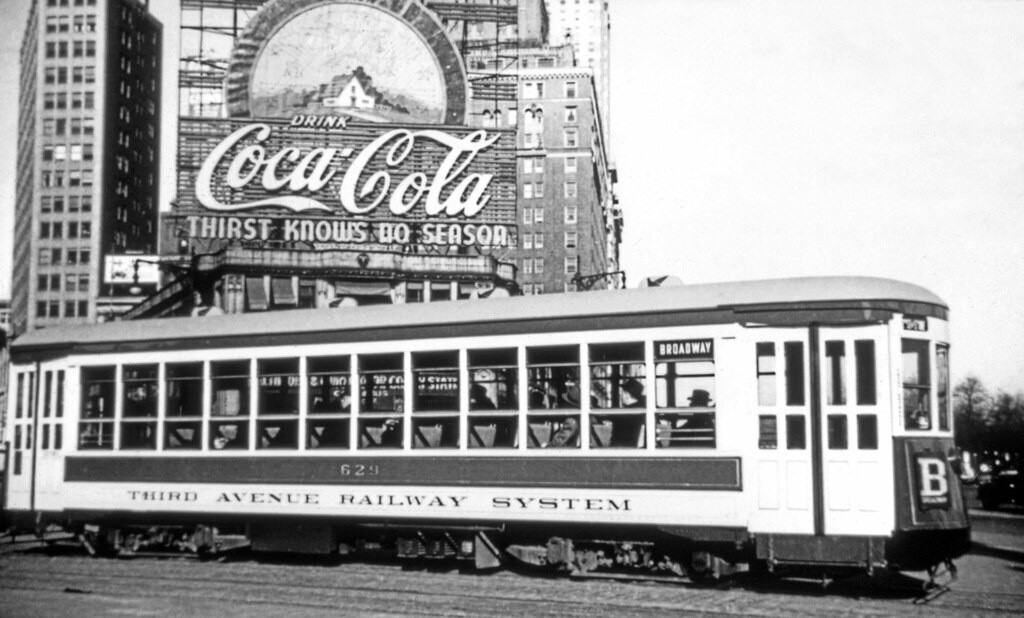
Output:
[619,378,647,445]
[669,390,715,447]
[470,385,496,427]
[528,393,547,425]
[916,412,930,428]
[317,393,351,448]
[541,386,580,450]
[382,398,404,446]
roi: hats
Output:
[561,385,580,407]
[687,389,713,402]
[616,377,644,401]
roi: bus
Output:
[0,275,972,595]
